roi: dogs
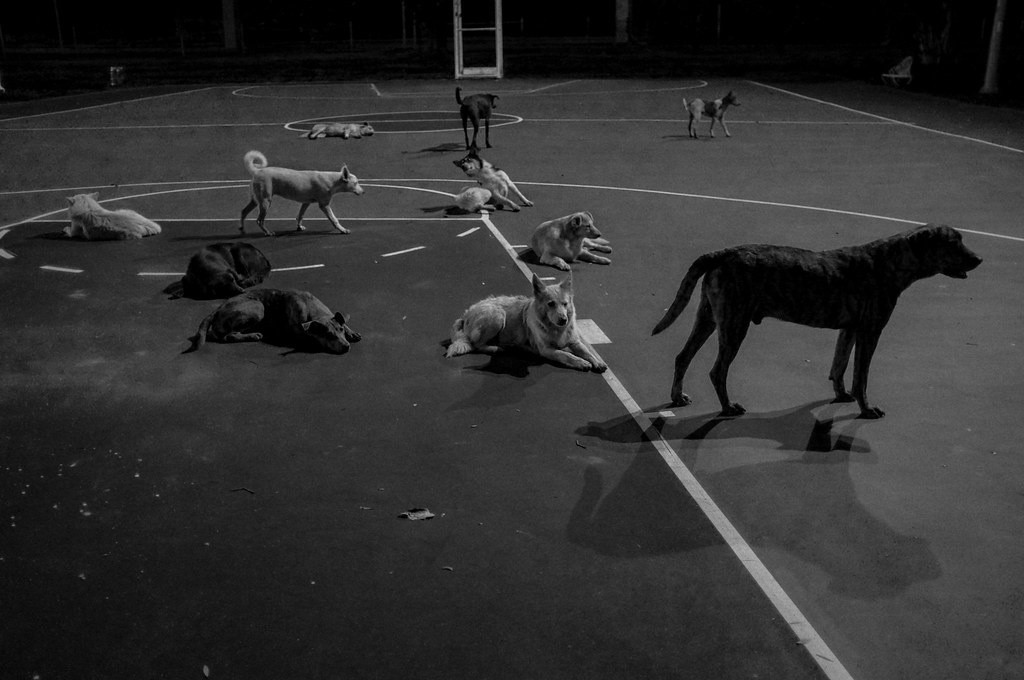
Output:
[531,211,612,270]
[62,191,162,239]
[683,88,741,137]
[652,224,983,419]
[181,289,362,353]
[297,122,374,139]
[452,148,534,212]
[238,151,364,234]
[456,87,500,150]
[447,270,608,373]
[163,242,271,299]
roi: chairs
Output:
[880,56,914,90]
